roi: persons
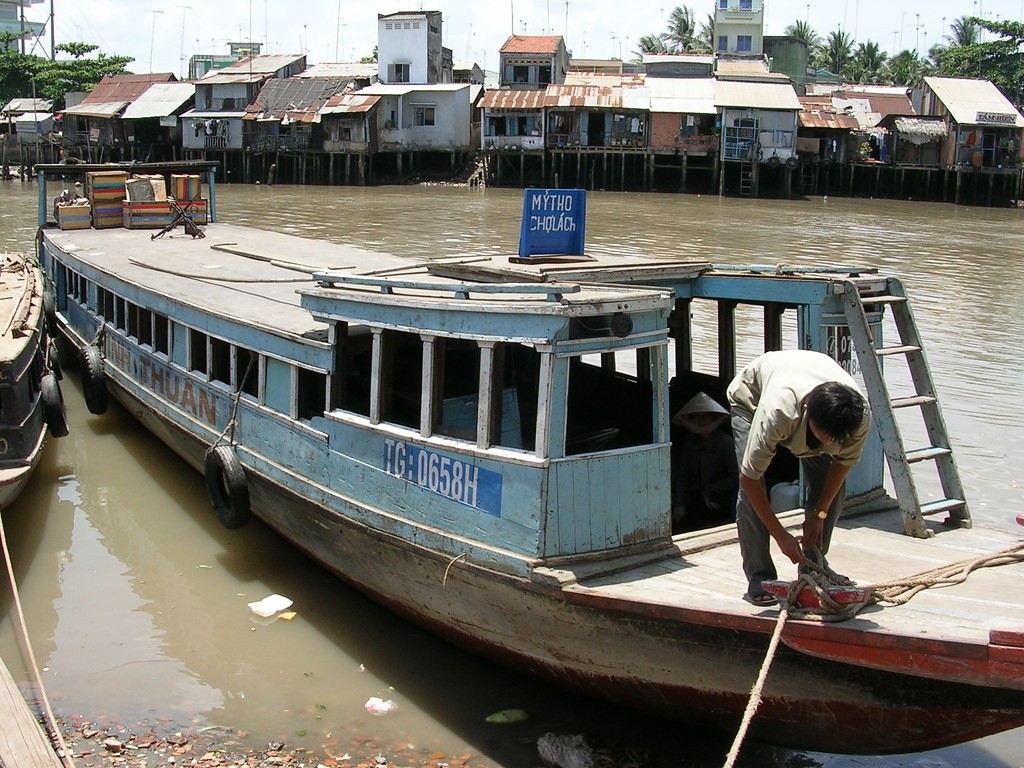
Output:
[1008,137,1017,169]
[727,350,872,606]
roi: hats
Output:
[671,392,731,426]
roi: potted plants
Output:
[488,139,494,149]
[504,144,509,149]
[512,143,516,149]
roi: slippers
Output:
[744,587,778,606]
[798,567,852,586]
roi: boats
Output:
[0,248,72,515]
[32,156,1023,702]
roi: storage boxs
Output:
[58,171,208,230]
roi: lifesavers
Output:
[751,141,762,151]
[786,157,798,169]
[752,151,763,162]
[41,370,70,438]
[47,348,64,381]
[202,445,250,531]
[79,345,110,415]
[768,157,780,168]
